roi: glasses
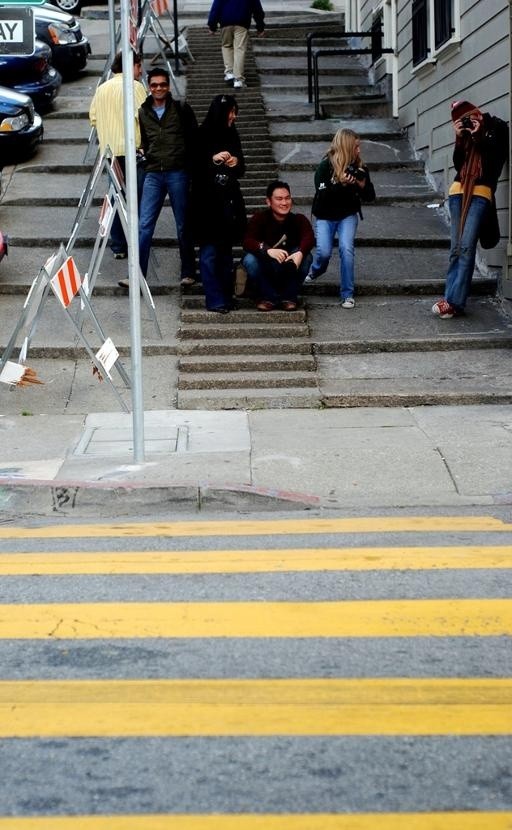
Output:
[149,82,168,89]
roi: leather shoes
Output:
[257,300,273,311]
[207,302,243,315]
[282,301,296,311]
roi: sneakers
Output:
[112,252,126,260]
[223,74,248,88]
[180,278,196,285]
[305,271,316,282]
[117,276,129,286]
[431,299,451,315]
[440,310,458,320]
[342,296,355,310]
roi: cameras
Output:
[344,164,365,181]
[457,115,473,134]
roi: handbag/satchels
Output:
[479,196,501,250]
[313,183,363,222]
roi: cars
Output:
[0,0,94,261]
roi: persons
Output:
[303,127,376,309]
[117,66,199,290]
[430,100,509,320]
[240,178,315,312]
[186,90,248,315]
[88,48,148,260]
[207,0,266,89]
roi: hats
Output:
[450,100,480,124]
